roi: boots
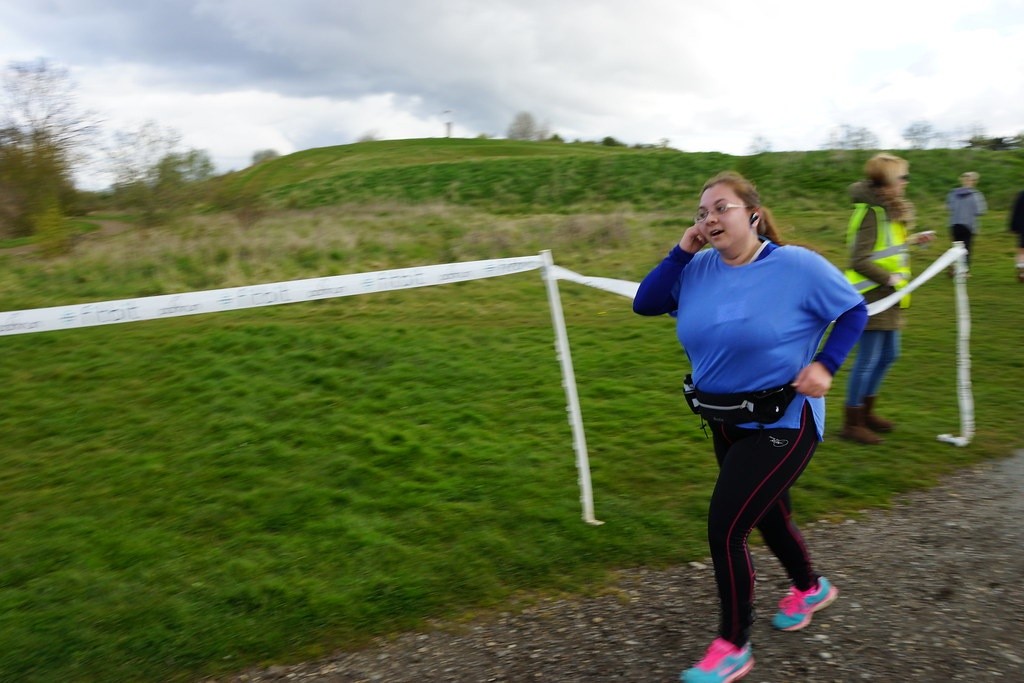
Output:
[842,406,881,444]
[864,396,894,430]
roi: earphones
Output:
[749,212,758,225]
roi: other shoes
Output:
[1017,265,1024,282]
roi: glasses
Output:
[898,174,909,181]
[694,203,749,220]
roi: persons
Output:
[1006,189,1024,282]
[945,170,987,279]
[633,170,868,683]
[844,152,936,444]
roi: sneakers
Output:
[771,576,839,631]
[680,637,756,683]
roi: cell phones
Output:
[909,231,937,241]
